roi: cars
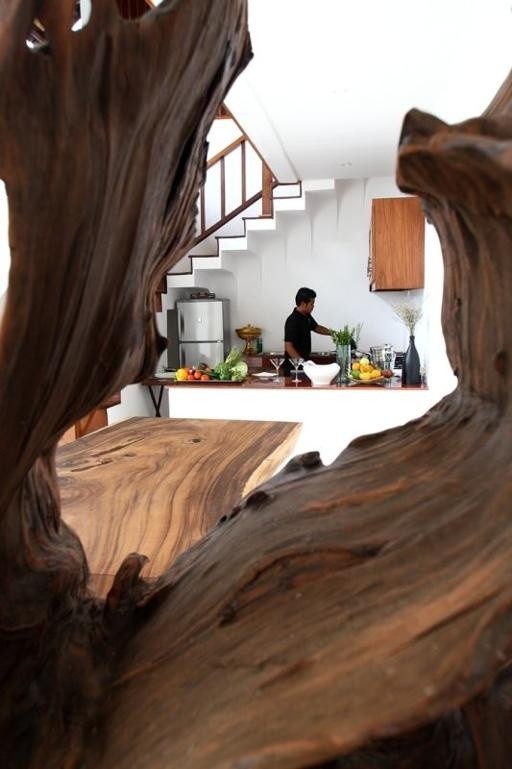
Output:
[336,344,351,384]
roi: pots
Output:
[369,344,396,372]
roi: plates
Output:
[367,197,425,292]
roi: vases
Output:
[391,302,424,335]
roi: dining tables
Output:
[328,324,356,384]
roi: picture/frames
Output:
[44,414,303,597]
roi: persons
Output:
[283,287,335,377]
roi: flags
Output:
[401,335,421,387]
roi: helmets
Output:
[346,373,385,384]
[252,372,279,380]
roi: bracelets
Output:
[303,364,341,385]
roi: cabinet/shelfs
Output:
[402,336,421,388]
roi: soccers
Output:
[187,375,194,380]
[351,358,381,380]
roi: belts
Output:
[288,358,304,382]
[235,323,262,356]
[270,358,285,383]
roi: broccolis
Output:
[176,299,224,370]
